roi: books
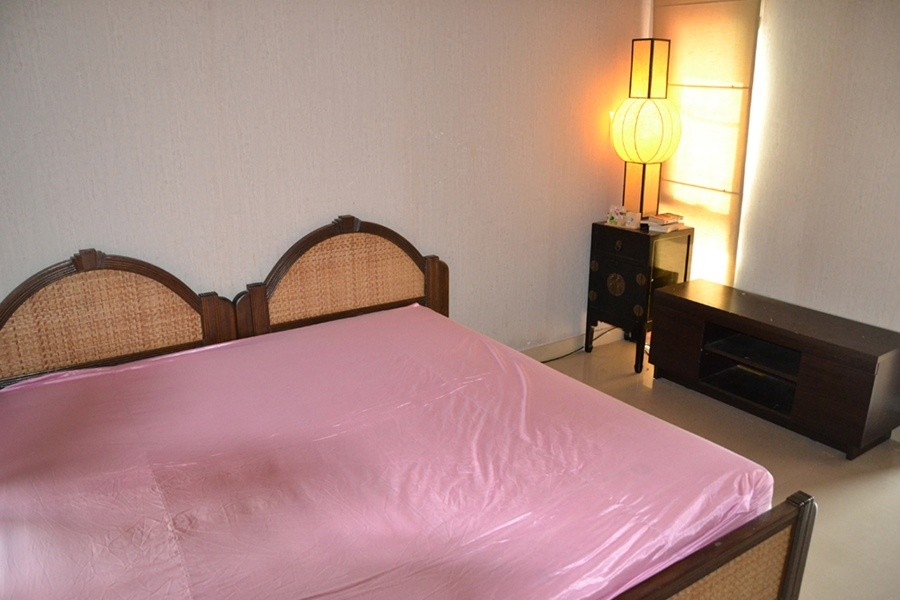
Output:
[649,212,683,224]
[648,222,684,233]
[641,221,677,226]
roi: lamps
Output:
[609,37,683,222]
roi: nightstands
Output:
[585,218,694,374]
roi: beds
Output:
[0,215,820,600]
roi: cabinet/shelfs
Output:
[647,278,900,462]
